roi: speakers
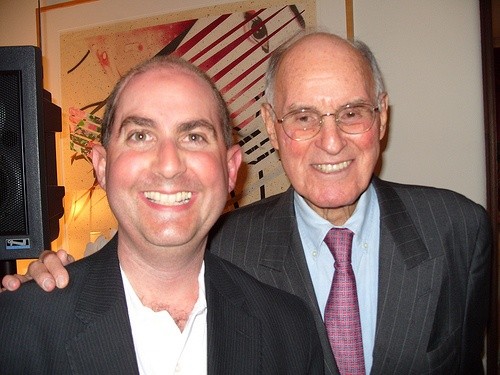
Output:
[0,46,65,261]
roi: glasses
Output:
[268,101,380,143]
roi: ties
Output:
[322,228,368,375]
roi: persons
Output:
[0,60,326,375]
[2,31,492,375]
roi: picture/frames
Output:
[34,1,354,268]
